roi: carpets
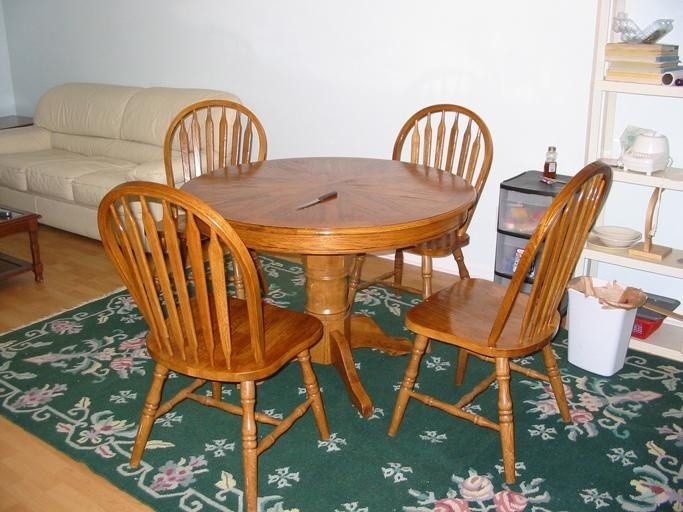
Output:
[0,251,683,512]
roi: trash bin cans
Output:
[565,275,647,376]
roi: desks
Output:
[174,158,476,416]
[0,116,34,131]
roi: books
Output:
[604,39,679,85]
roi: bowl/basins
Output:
[589,225,643,248]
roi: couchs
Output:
[0,83,242,256]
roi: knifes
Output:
[296,190,337,210]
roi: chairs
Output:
[346,103,494,353]
[98,182,329,511]
[144,101,268,302]
[390,160,613,483]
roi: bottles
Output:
[544,146,556,178]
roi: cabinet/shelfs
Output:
[494,170,573,315]
[565,0,683,365]
